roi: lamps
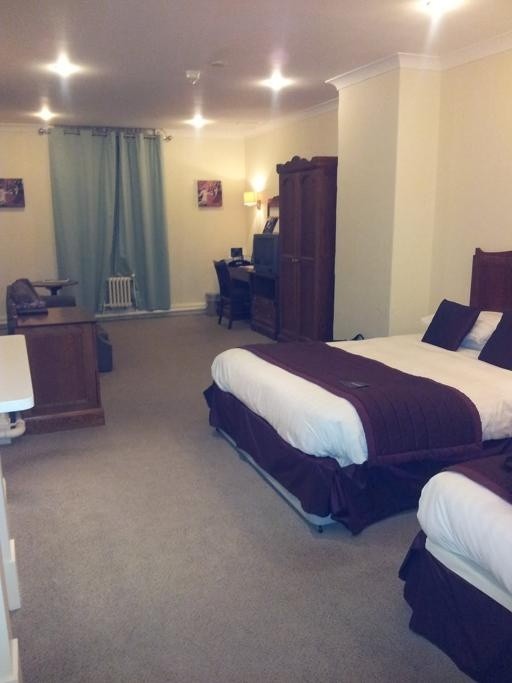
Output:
[243,165,271,207]
[0,332,35,446]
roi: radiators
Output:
[103,277,133,308]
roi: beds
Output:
[202,245,512,539]
[396,453,511,683]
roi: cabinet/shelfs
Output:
[277,154,340,345]
[14,305,105,436]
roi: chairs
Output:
[212,259,251,329]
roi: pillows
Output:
[420,299,481,352]
[422,307,503,350]
[477,311,512,371]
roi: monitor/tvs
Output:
[253,234,279,264]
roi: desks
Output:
[230,264,279,343]
[32,279,78,296]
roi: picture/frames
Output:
[0,178,25,210]
[196,181,223,208]
